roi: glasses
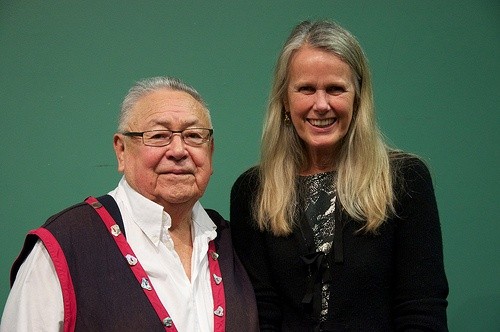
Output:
[122,128,213,147]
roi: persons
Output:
[230,20,450,332]
[0,76,261,332]
